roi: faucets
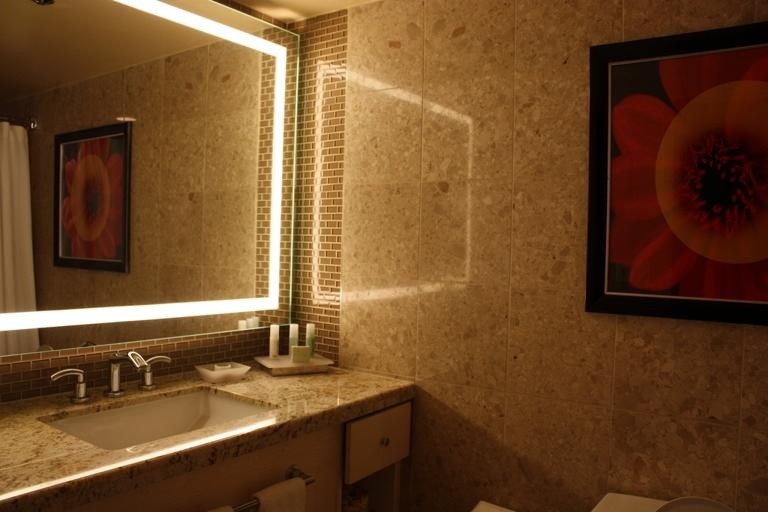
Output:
[82,340,95,346]
[104,350,141,398]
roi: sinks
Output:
[34,381,283,451]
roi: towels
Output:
[248,476,308,512]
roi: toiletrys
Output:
[268,324,281,360]
[288,323,299,357]
[306,323,316,359]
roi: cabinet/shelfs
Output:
[340,402,412,512]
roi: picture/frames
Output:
[52,121,133,276]
[584,22,768,327]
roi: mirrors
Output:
[0,1,302,360]
